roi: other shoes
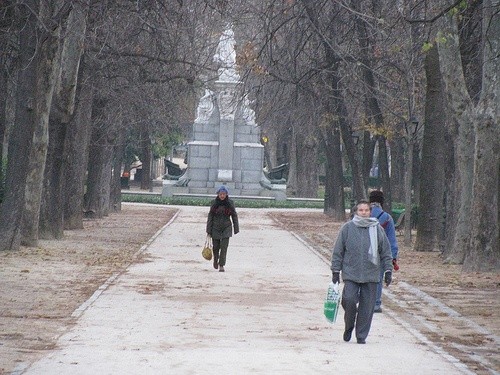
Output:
[220,268,224,272]
[357,338,365,343]
[344,331,351,341]
[214,263,218,269]
[374,306,382,312]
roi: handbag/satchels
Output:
[202,235,212,260]
[324,281,340,324]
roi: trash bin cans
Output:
[391,208,406,228]
[120,176,131,189]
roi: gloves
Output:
[332,272,340,284]
[385,272,391,285]
[392,260,399,270]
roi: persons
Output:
[355,189,398,314]
[329,199,394,344]
[206,187,240,274]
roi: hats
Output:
[216,186,228,194]
[370,191,384,209]
